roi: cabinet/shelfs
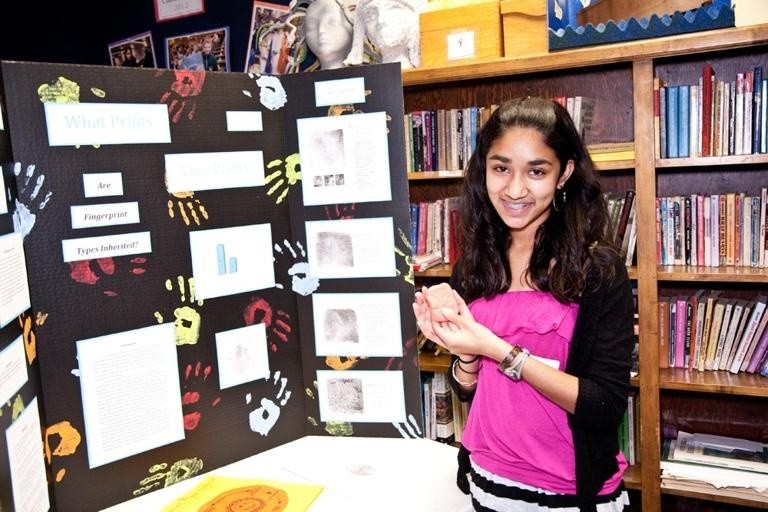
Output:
[402,24,768,512]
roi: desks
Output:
[97,435,476,512]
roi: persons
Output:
[114,9,297,74]
[301,1,353,70]
[358,1,420,70]
[409,93,636,512]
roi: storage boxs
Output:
[500,0,549,58]
[419,0,504,67]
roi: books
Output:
[410,190,636,267]
[653,64,768,159]
[659,292,768,377]
[657,188,768,266]
[661,430,768,488]
[421,374,635,465]
[409,96,635,172]
